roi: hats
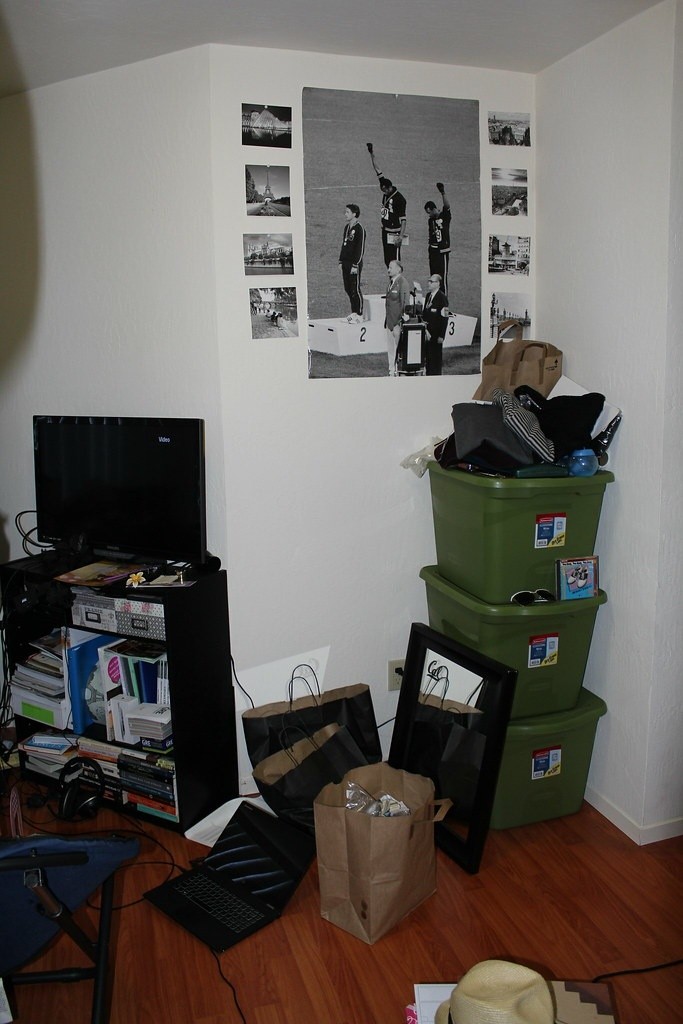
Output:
[434,959,558,1024]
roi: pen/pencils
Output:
[103,568,142,581]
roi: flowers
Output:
[126,571,146,588]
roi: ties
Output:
[428,296,432,305]
[390,279,393,287]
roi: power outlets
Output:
[387,659,405,692]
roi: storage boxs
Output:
[419,460,616,831]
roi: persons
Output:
[338,204,367,324]
[367,143,407,298]
[424,182,452,297]
[384,260,410,376]
[422,274,450,376]
[250,300,283,329]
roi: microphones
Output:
[47,805,65,821]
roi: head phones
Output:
[55,757,106,820]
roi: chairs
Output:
[0,829,140,1024]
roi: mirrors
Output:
[388,620,519,876]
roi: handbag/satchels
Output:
[250,722,370,835]
[449,400,543,471]
[403,668,487,812]
[313,762,452,945]
[241,664,383,768]
[472,319,563,404]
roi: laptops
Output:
[143,801,317,953]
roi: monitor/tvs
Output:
[32,414,206,571]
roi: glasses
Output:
[428,280,437,284]
[510,587,556,606]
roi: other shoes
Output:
[347,313,364,323]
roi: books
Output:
[10,626,179,823]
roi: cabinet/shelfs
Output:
[0,547,239,837]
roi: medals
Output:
[344,243,346,246]
[381,210,384,215]
